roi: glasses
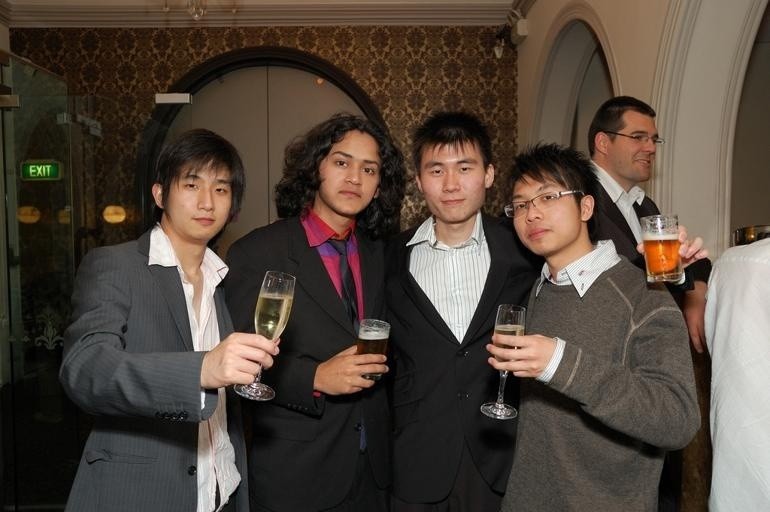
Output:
[603,131,665,146]
[504,190,585,218]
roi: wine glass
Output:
[233,269,295,403]
[479,302,525,421]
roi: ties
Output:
[325,229,359,325]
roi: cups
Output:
[640,212,684,284]
[356,318,391,382]
